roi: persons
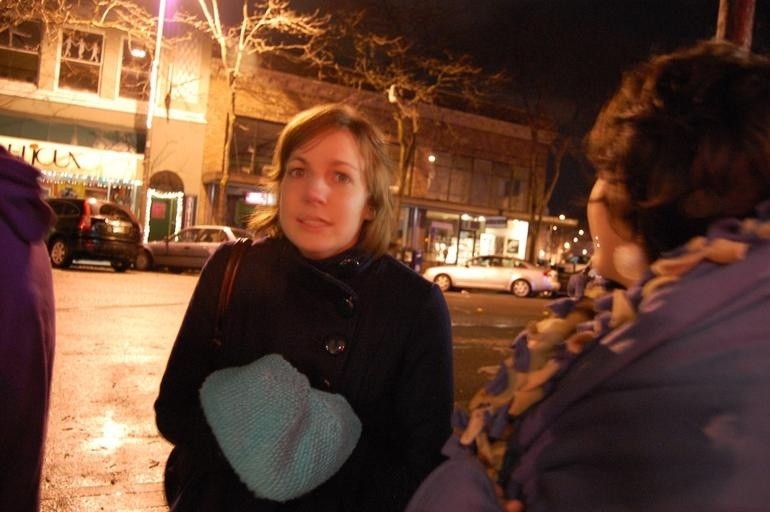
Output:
[391,34,770,512]
[1,139,58,512]
[150,101,455,512]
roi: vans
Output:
[47,197,143,273]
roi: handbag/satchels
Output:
[164,238,253,512]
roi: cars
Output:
[423,254,562,298]
[135,224,253,271]
[554,256,592,276]
